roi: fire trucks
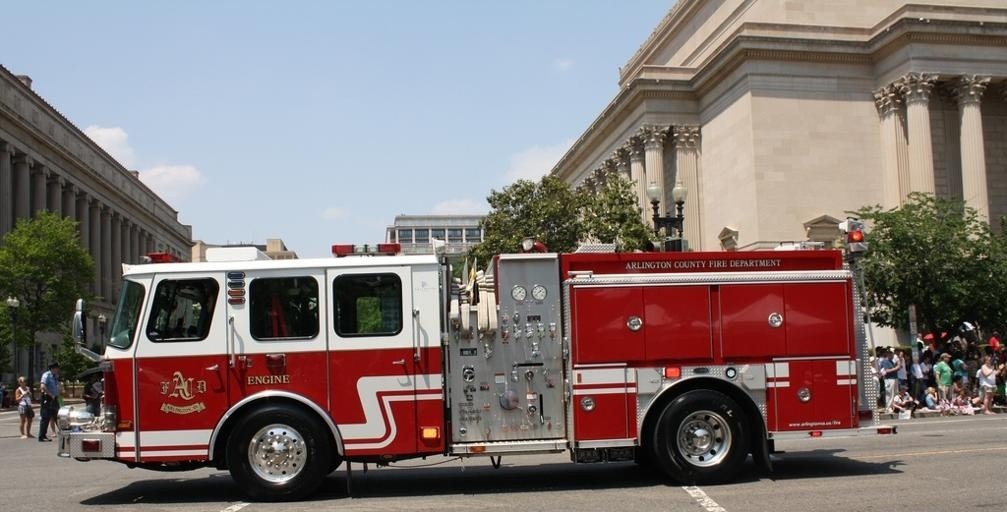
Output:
[51,237,901,502]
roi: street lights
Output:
[97,311,106,356]
[5,291,22,400]
[647,179,691,251]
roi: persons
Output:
[869,331,1007,415]
[83,376,101,417]
[37,363,61,441]
[206,294,216,313]
[49,381,65,437]
[15,376,35,439]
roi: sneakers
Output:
[22,433,56,441]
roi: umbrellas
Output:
[77,367,104,384]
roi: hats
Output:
[47,362,60,369]
[941,352,952,358]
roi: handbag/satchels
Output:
[26,405,34,418]
